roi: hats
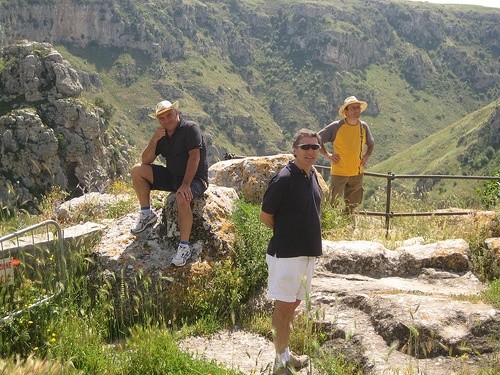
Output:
[339,96,368,118]
[148,100,180,119]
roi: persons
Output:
[131,101,209,267]
[260,128,323,375]
[316,96,374,230]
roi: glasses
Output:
[297,144,320,150]
[348,107,361,110]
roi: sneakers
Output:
[170,245,191,266]
[130,210,159,233]
[273,353,308,375]
